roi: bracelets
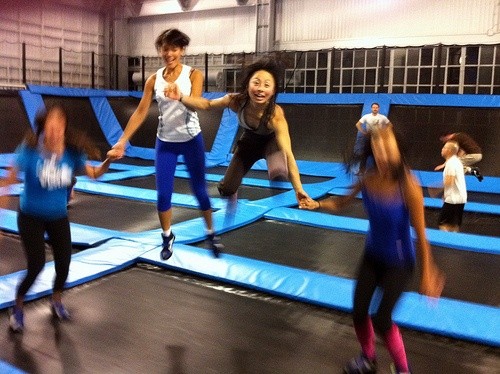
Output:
[179,92,183,102]
[317,200,320,209]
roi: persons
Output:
[0,97,112,332]
[356,103,394,176]
[105,28,225,260]
[162,55,312,209]
[295,126,447,374]
[440,132,484,182]
[428,140,467,233]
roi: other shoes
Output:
[50,301,70,319]
[343,353,377,374]
[354,171,364,176]
[9,309,24,331]
[471,168,484,182]
[390,362,413,374]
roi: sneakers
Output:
[161,230,175,261]
[206,233,224,258]
